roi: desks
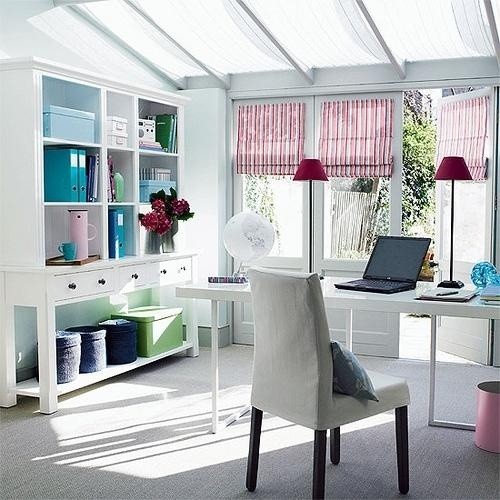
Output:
[175,274,500,435]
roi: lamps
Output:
[435,156,474,288]
[293,159,329,280]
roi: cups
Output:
[58,243,78,260]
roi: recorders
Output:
[139,118,155,142]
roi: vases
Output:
[152,231,174,253]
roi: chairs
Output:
[243,267,411,499]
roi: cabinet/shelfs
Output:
[0,56,200,414]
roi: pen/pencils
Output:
[437,292,459,295]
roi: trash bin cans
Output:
[474,381,500,453]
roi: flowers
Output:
[138,187,195,236]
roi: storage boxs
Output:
[111,305,184,358]
[140,181,177,202]
[107,116,128,135]
[98,319,138,364]
[65,325,107,373]
[36,332,82,384]
[107,133,129,147]
[43,103,95,144]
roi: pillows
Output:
[327,339,380,405]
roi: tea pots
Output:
[67,209,98,260]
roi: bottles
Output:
[114,172,126,202]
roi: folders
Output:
[44,149,86,202]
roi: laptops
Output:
[335,236,431,294]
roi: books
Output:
[139,112,177,154]
[414,284,500,305]
